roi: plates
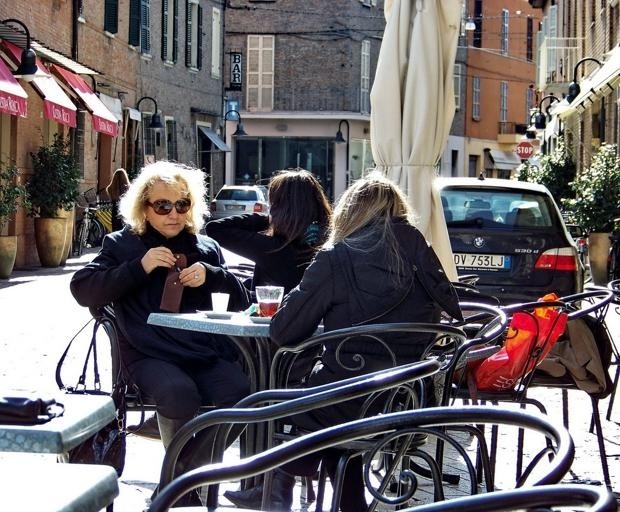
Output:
[205,312,235,319]
[249,317,274,323]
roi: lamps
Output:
[535,96,560,129]
[566,58,603,105]
[526,111,546,139]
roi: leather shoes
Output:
[127,412,160,439]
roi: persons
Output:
[223,171,466,511]
[205,165,333,303]
[69,155,250,508]
[105,166,132,232]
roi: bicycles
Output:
[74,185,114,256]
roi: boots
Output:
[150,410,249,508]
[223,467,296,512]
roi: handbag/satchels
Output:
[0,388,65,425]
[57,390,126,478]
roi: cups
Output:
[256,286,285,317]
[211,293,230,313]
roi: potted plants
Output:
[0,130,82,279]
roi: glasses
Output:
[146,197,191,215]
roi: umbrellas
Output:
[368,0,466,287]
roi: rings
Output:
[193,272,199,279]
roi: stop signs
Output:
[515,141,535,161]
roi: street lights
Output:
[132,96,168,183]
[331,119,350,192]
[223,108,248,186]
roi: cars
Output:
[432,168,588,310]
[208,184,270,219]
